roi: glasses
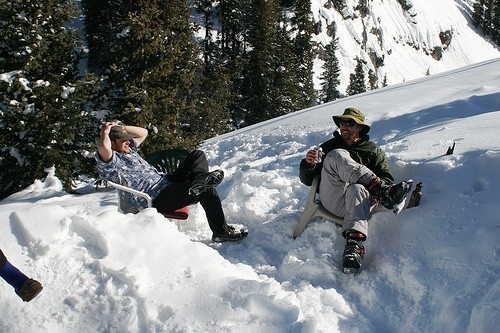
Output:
[340,119,357,127]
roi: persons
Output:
[0,250,43,302]
[299,108,413,271]
[95,119,248,241]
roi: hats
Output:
[332,108,370,135]
[109,124,133,141]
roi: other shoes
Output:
[14,278,43,303]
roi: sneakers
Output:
[212,226,248,242]
[384,179,413,213]
[188,170,224,196]
[342,242,362,270]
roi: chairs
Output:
[144,148,192,175]
[99,175,190,222]
[292,170,377,241]
